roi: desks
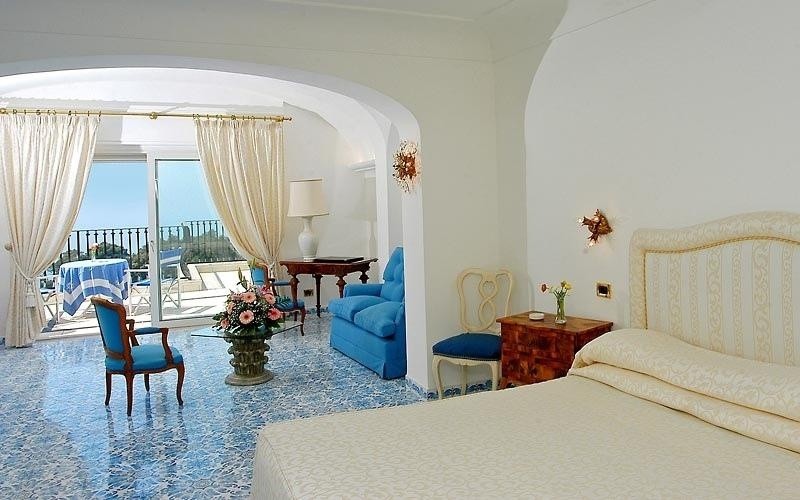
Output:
[58,259,133,319]
[280,258,379,319]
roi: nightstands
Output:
[494,309,612,388]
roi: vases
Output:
[90,252,95,262]
[553,297,567,324]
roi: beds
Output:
[249,205,800,497]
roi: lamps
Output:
[288,177,329,263]
[578,210,614,248]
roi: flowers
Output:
[542,281,573,320]
[88,242,100,255]
[216,257,303,330]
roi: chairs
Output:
[89,297,185,417]
[327,247,408,380]
[432,269,516,400]
[35,275,62,324]
[124,248,187,318]
[246,258,306,336]
